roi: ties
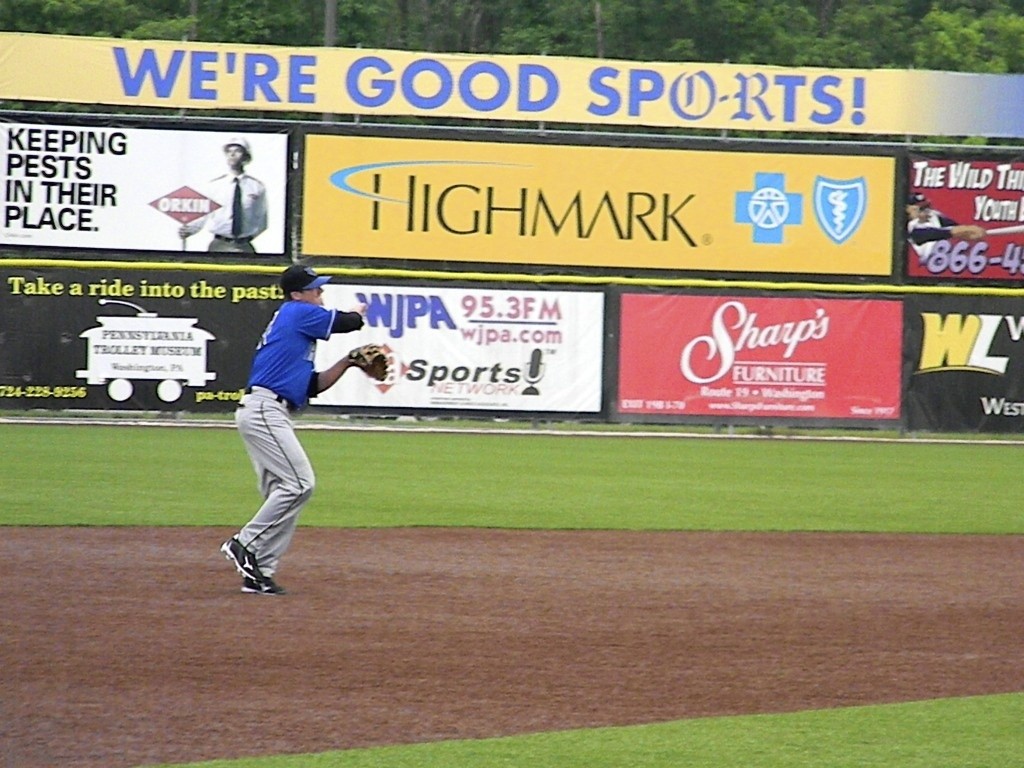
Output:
[232,178,243,235]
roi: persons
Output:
[178,138,269,256]
[220,264,388,597]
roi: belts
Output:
[214,234,252,242]
[245,387,297,412]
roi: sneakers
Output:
[221,537,265,584]
[241,577,287,596]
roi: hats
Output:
[908,191,932,207]
[279,265,335,291]
[224,136,252,164]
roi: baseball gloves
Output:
[347,343,388,384]
